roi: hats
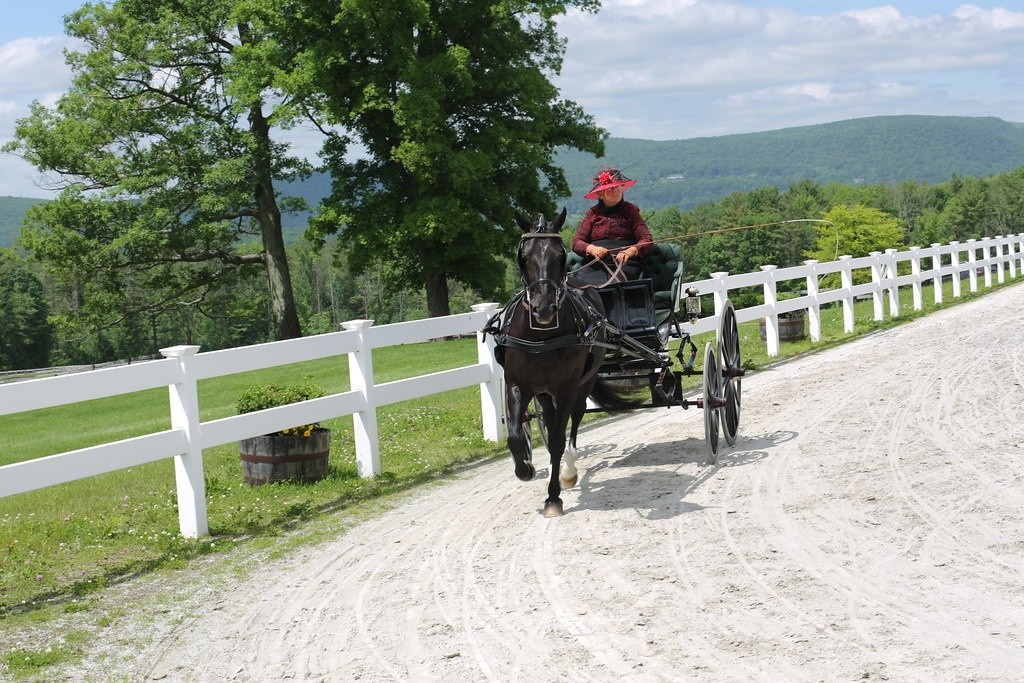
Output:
[584,169,636,199]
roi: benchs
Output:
[566,243,684,326]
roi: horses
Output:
[503,206,649,518]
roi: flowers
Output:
[756,291,805,321]
[234,374,326,437]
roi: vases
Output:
[238,428,331,486]
[759,317,804,344]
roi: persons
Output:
[571,166,654,285]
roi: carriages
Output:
[480,205,748,519]
